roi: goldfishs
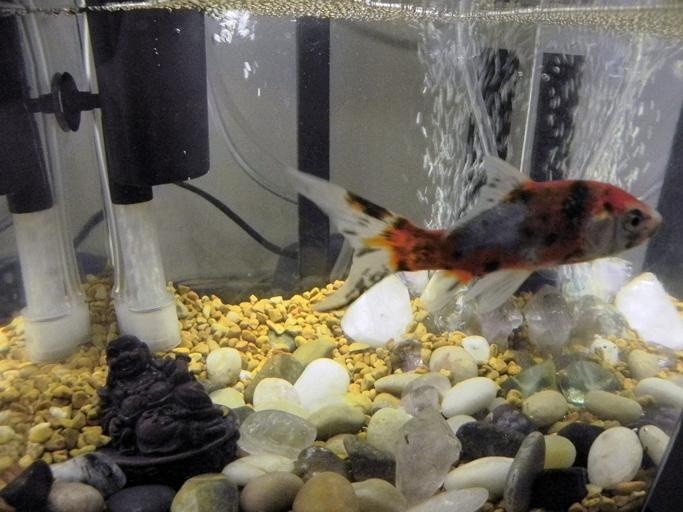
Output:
[279,154,665,320]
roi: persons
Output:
[88,330,227,450]
